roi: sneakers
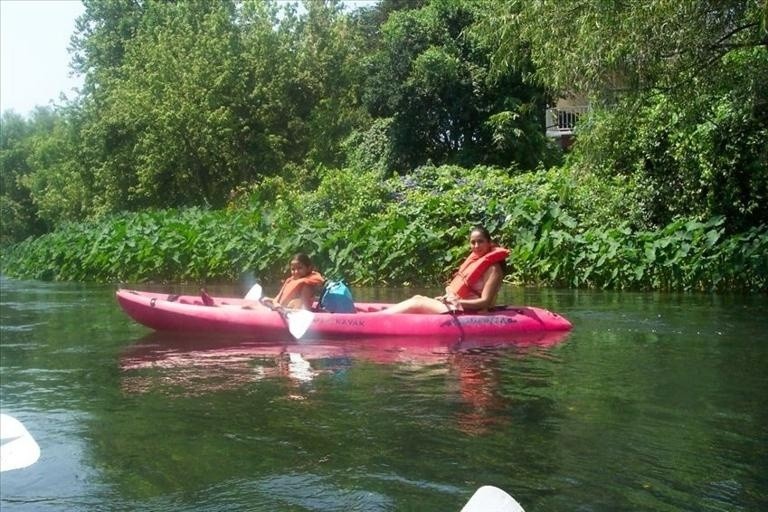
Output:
[201,288,214,306]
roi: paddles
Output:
[444,302,463,330]
[243,283,315,339]
[1,415,40,473]
[461,485,524,511]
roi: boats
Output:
[115,288,572,337]
[117,333,567,370]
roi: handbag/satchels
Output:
[320,279,353,313]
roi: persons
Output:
[199,253,316,310]
[361,224,506,316]
